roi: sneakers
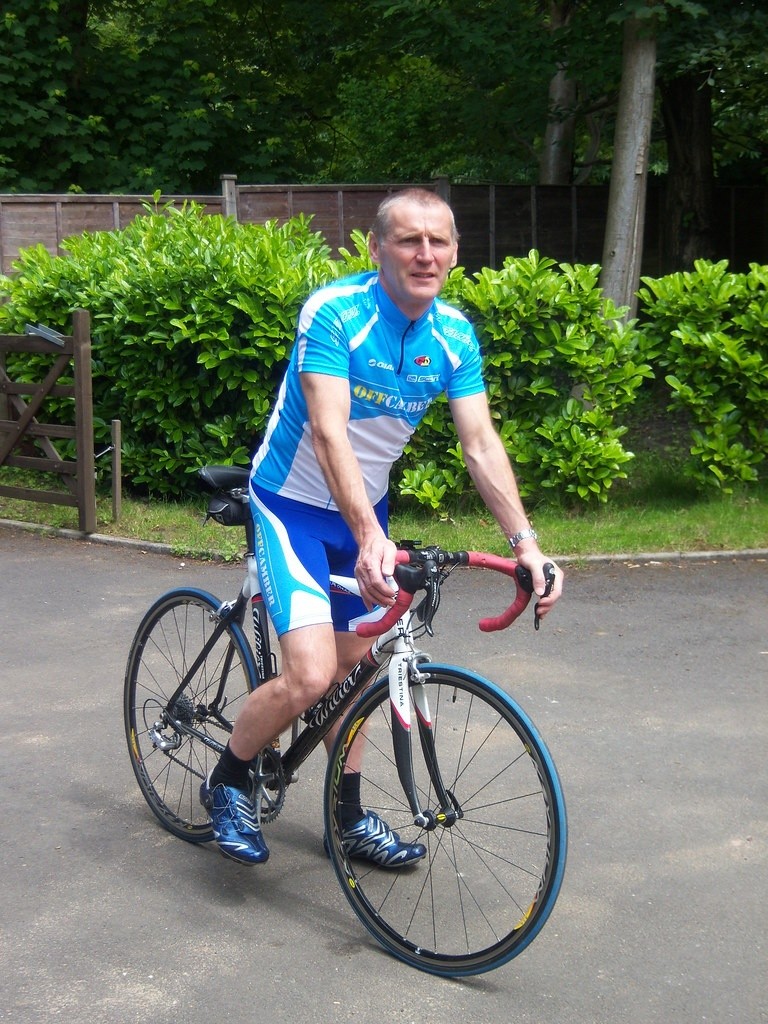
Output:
[324,810,427,867]
[198,768,270,867]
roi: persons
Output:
[195,188,565,867]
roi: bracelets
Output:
[508,529,539,550]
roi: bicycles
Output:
[123,464,568,979]
